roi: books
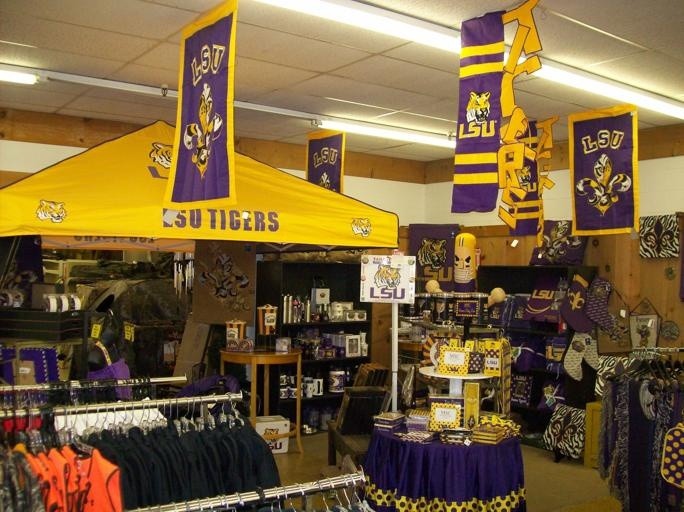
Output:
[470,425,506,446]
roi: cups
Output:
[410,327,426,343]
[280,375,324,398]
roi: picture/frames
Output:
[345,334,361,358]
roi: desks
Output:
[220,346,305,455]
[371,365,522,512]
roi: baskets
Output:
[1,346,60,409]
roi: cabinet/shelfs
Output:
[477,262,600,451]
[251,254,391,432]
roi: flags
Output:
[569,105,641,235]
[166,2,237,208]
[305,129,348,197]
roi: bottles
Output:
[293,300,299,323]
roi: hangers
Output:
[207,469,374,512]
[0,377,245,451]
[599,347,684,381]
